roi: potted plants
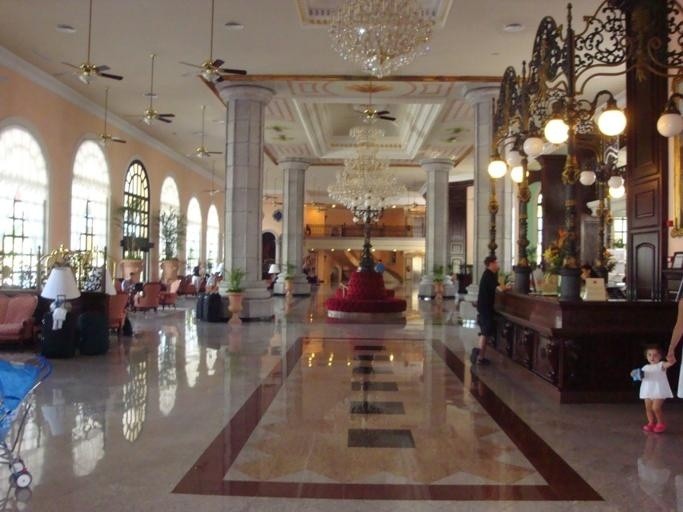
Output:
[430,274,446,303]
[152,201,192,284]
[278,261,296,295]
[110,190,153,285]
[222,261,247,326]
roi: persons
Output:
[444,272,469,322]
[191,261,201,294]
[121,271,137,313]
[468,362,495,478]
[627,341,676,434]
[466,254,512,367]
[664,279,682,402]
[579,264,592,282]
[373,259,383,275]
[629,429,671,512]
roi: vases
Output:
[538,266,558,297]
[582,274,608,305]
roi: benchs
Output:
[334,286,395,302]
[322,292,408,324]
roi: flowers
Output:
[574,247,617,283]
[531,226,568,271]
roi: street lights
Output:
[353,193,385,272]
[347,356,381,432]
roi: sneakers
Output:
[478,359,491,366]
[641,420,656,433]
[654,421,667,434]
[469,347,479,364]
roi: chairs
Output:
[0,262,222,363]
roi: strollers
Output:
[0,351,52,489]
[0,485,32,512]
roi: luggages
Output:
[41,305,78,359]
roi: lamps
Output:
[315,2,441,228]
[483,80,682,202]
[265,262,282,290]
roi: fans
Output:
[49,0,124,88]
[79,85,127,146]
[261,167,284,210]
[199,159,224,199]
[179,102,223,161]
[121,50,177,129]
[175,0,249,84]
